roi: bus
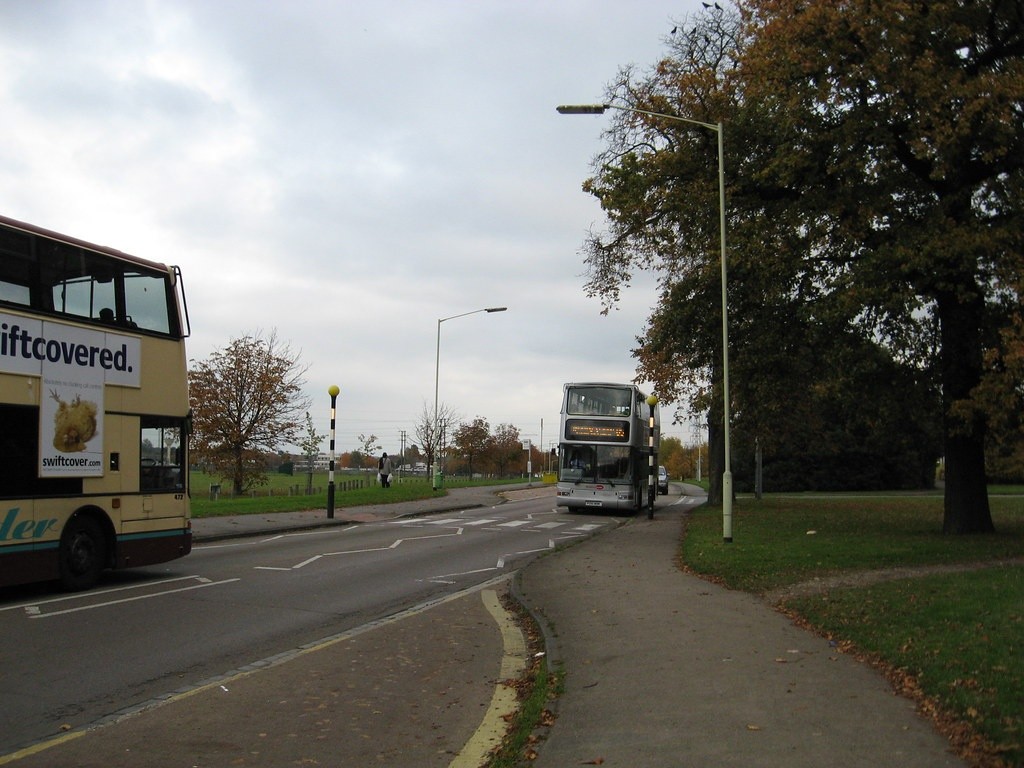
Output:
[0,215,197,584]
[560,381,661,513]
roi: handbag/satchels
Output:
[376,472,382,483]
[387,473,393,483]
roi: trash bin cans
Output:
[435,472,444,489]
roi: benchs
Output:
[91,316,137,329]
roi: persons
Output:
[100,308,114,321]
[378,452,391,488]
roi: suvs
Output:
[657,465,670,496]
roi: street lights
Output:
[429,306,508,492]
[324,384,342,517]
[556,102,733,540]
[646,394,660,518]
